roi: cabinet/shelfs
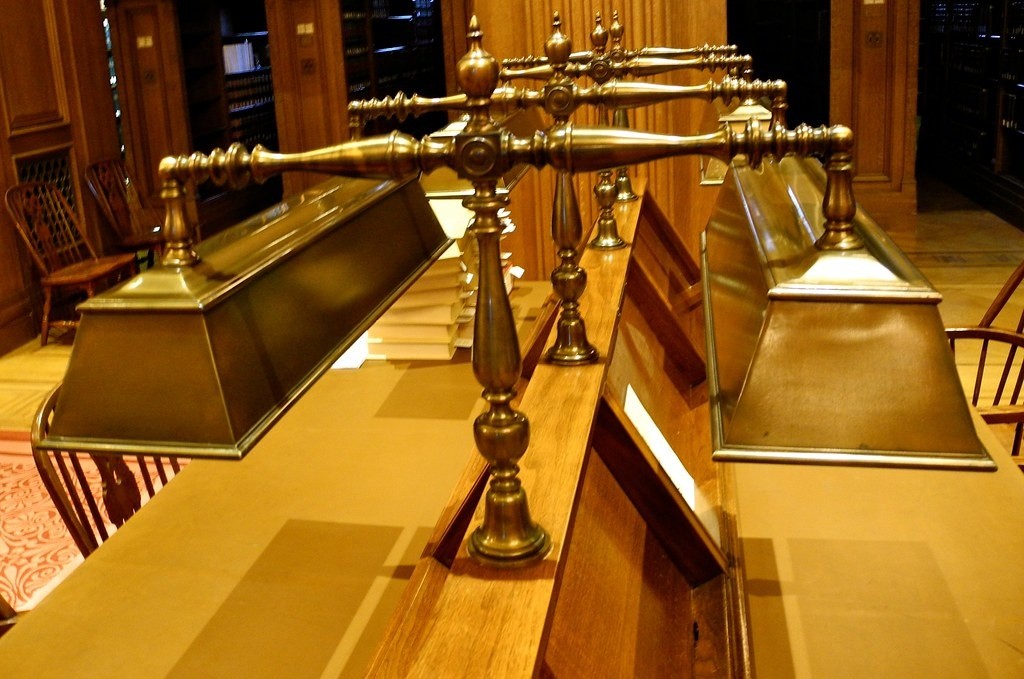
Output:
[921,0,1023,231]
[102,0,438,220]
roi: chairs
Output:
[83,157,199,275]
[944,260,1024,456]
[5,180,139,347]
[33,381,178,561]
